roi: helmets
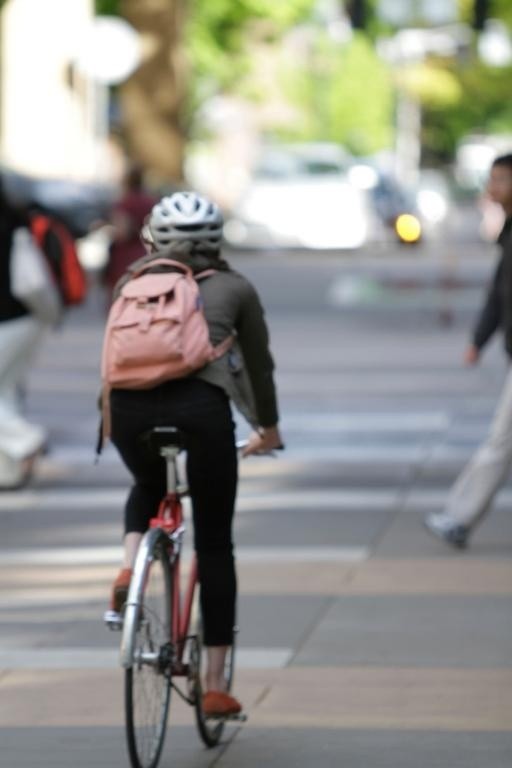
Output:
[139,192,224,257]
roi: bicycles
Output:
[118,428,285,768]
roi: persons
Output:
[89,170,159,319]
[96,188,283,721]
[1,171,63,491]
[420,152,512,551]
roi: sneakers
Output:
[104,570,144,620]
[423,514,469,547]
[203,692,241,715]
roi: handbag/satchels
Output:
[11,228,65,322]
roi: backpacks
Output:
[102,259,236,390]
[23,200,84,306]
